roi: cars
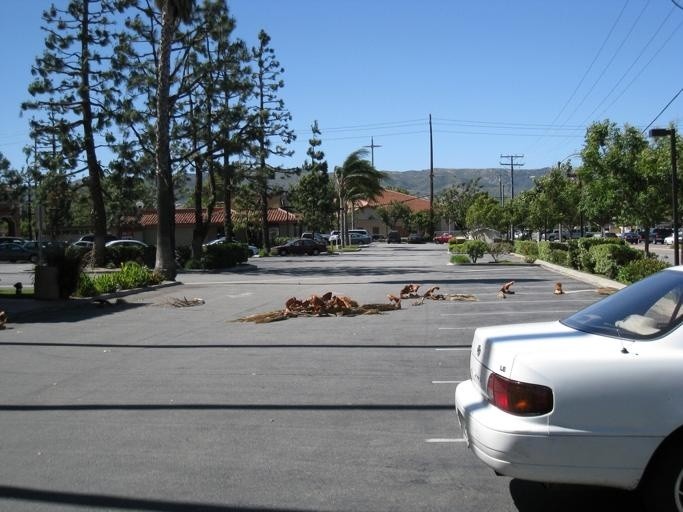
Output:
[0,234,150,262]
[202,235,259,257]
[514,227,682,244]
[454,265,683,512]
[270,236,326,256]
[407,232,423,243]
[388,230,401,243]
[301,228,371,244]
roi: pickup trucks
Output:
[432,232,453,244]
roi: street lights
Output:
[649,125,680,264]
